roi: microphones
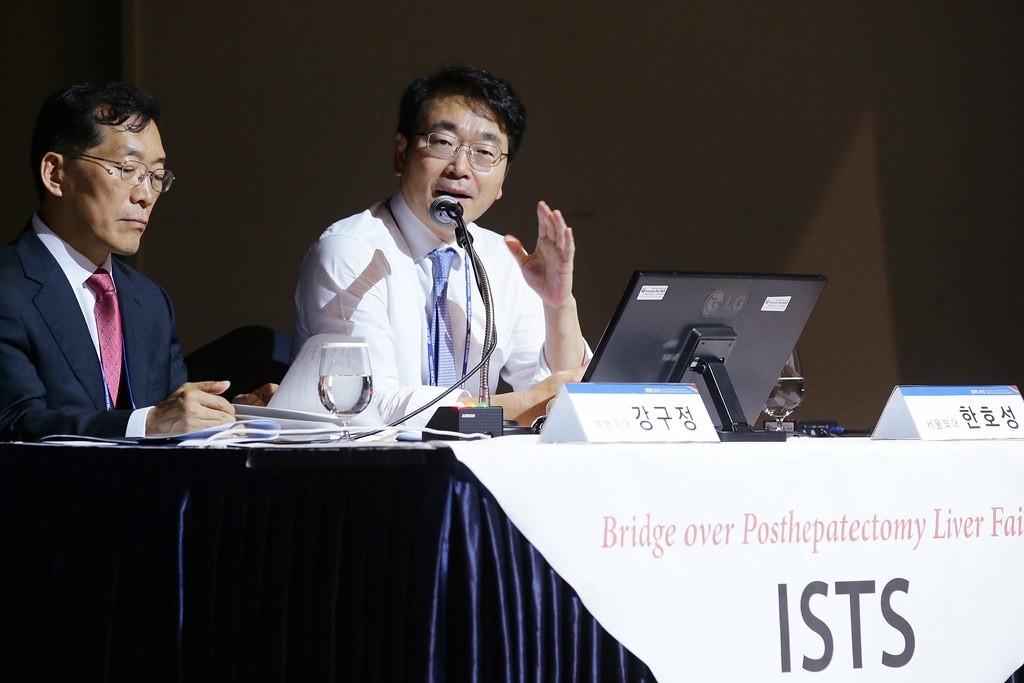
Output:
[429,195,463,225]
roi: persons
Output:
[292,66,594,427]
[0,80,279,441]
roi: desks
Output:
[0,434,1024,683]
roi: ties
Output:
[428,249,459,386]
[82,268,124,408]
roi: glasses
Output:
[57,148,176,193]
[411,131,510,168]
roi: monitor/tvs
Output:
[581,271,828,442]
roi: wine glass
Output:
[762,347,805,431]
[318,342,373,444]
